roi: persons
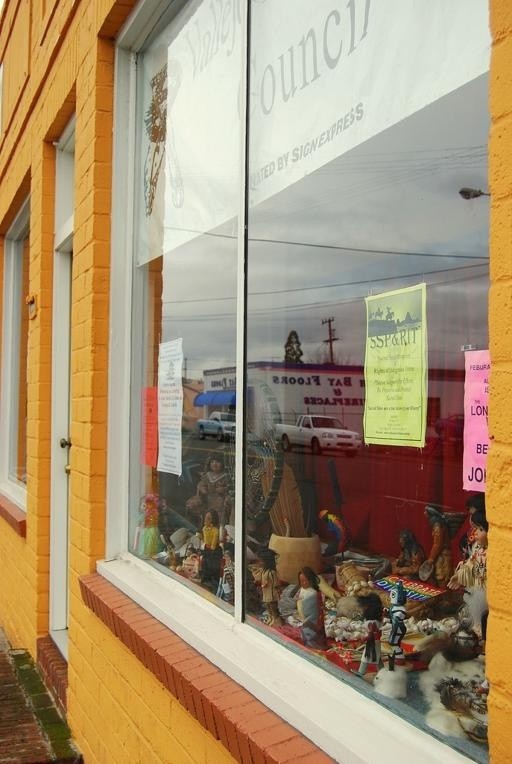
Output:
[140,453,234,602]
[293,569,326,647]
[139,452,488,678]
[351,582,408,677]
[257,552,283,626]
[394,495,487,637]
[319,509,350,554]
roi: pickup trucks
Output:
[196,410,236,441]
[274,414,361,457]
